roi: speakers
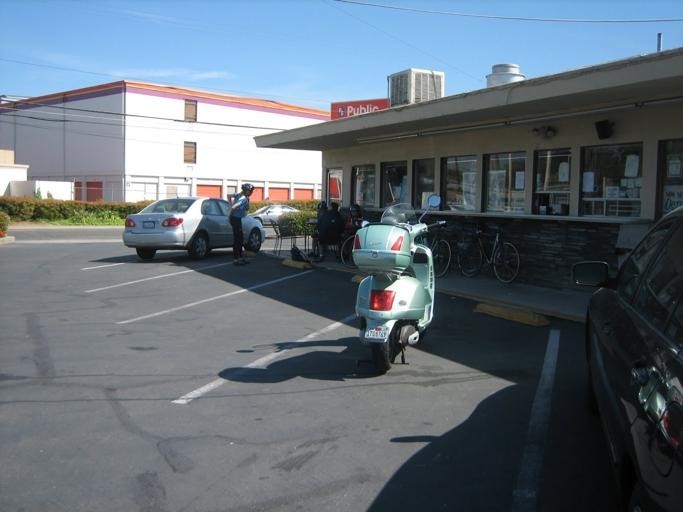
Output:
[595,119,614,139]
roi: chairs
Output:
[269,217,342,259]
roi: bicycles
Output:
[340,220,519,284]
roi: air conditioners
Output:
[387,67,444,109]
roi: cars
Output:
[248,204,303,227]
[573,205,682,512]
[122,197,266,259]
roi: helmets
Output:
[317,201,328,210]
[242,183,254,192]
[349,204,360,211]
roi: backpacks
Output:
[289,244,308,262]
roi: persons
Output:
[227,183,254,265]
[309,201,364,263]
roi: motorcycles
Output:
[351,194,445,374]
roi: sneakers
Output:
[233,260,245,265]
[239,258,249,264]
